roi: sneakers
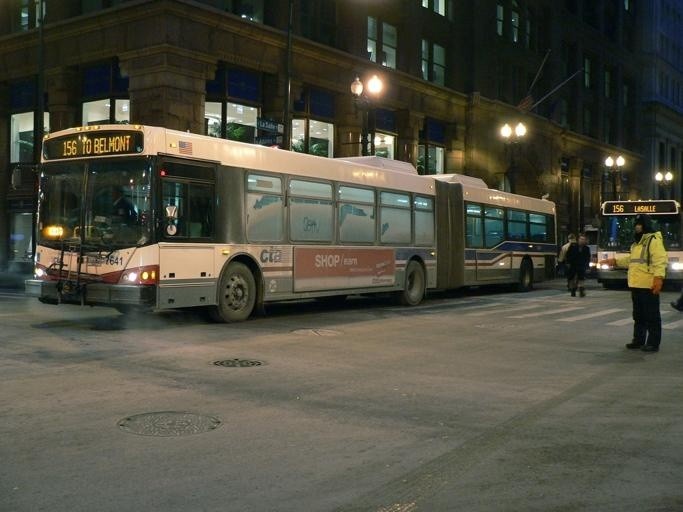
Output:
[670,301,683,312]
[626,343,658,352]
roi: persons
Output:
[671,292,682,312]
[567,236,591,297]
[626,212,667,351]
[111,185,138,223]
[58,179,77,215]
[559,233,579,292]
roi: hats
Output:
[634,213,650,231]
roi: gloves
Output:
[651,277,663,294]
[600,258,615,267]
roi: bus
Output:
[593,197,683,289]
[27,121,560,329]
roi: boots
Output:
[570,287,586,298]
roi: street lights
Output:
[499,121,531,197]
[653,170,678,201]
[603,153,629,202]
[349,68,394,158]
